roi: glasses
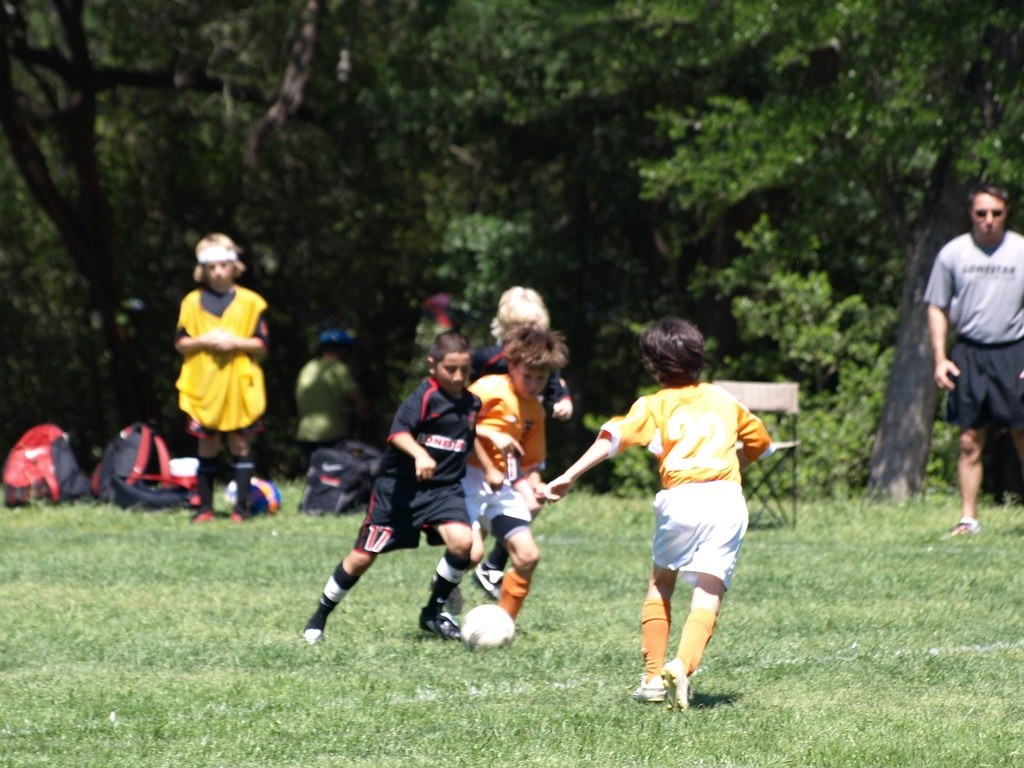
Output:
[973,205,1005,216]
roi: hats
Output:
[319,326,355,344]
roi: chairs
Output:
[711,382,801,534]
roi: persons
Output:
[923,183,1024,541]
[294,327,366,469]
[302,330,482,646]
[428,324,569,621]
[174,233,267,524]
[543,318,775,712]
[467,285,573,599]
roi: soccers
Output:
[460,604,514,650]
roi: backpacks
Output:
[3,425,91,508]
[93,423,170,504]
[299,441,385,517]
[112,472,201,510]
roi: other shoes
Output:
[950,521,980,535]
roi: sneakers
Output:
[472,561,504,601]
[303,619,325,643]
[419,606,460,640]
[659,657,689,711]
[442,584,463,616]
[189,506,212,522]
[633,674,667,703]
[231,509,245,523]
